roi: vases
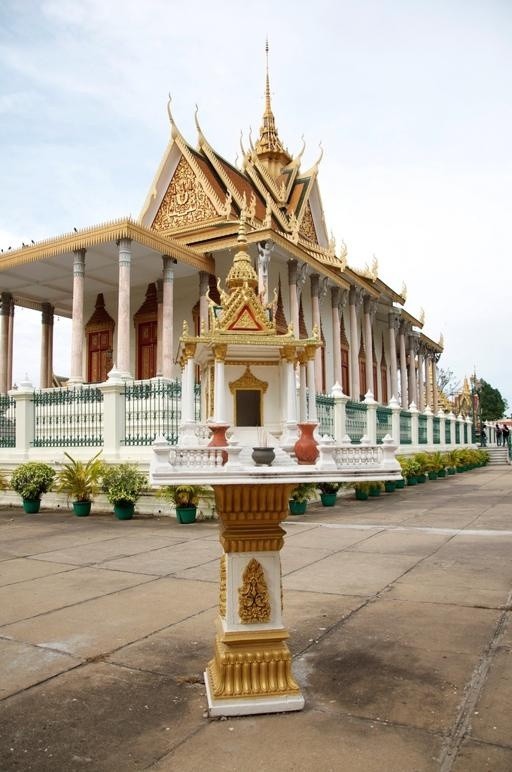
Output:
[384,481,395,492]
[294,423,320,464]
[208,426,230,466]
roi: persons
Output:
[495,423,509,447]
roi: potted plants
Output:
[290,480,381,515]
[10,448,213,524]
[395,447,491,488]
